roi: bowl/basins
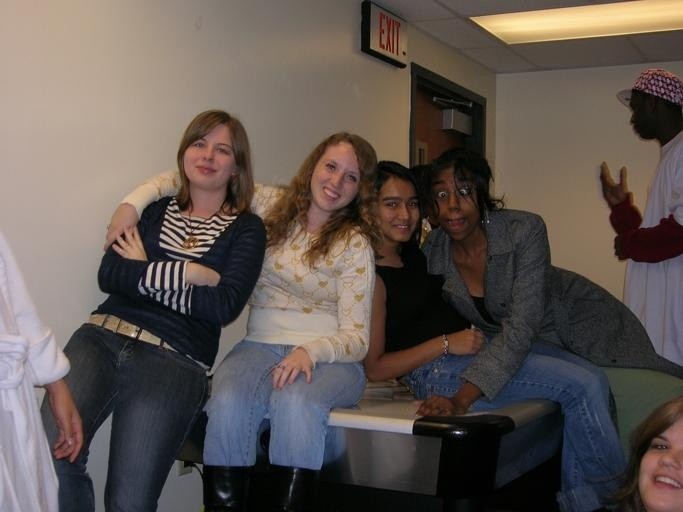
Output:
[182,202,231,249]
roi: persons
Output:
[363,159,628,512]
[600,395,683,512]
[0,231,84,512]
[39,109,267,511]
[102,131,381,512]
[599,68,682,367]
[413,146,682,418]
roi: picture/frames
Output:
[202,397,566,512]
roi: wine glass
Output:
[278,364,285,369]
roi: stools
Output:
[88,314,180,355]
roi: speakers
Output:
[204,466,246,511]
[270,466,314,512]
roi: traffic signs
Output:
[177,461,193,477]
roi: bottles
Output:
[617,67,682,107]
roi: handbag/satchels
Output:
[441,333,450,358]
